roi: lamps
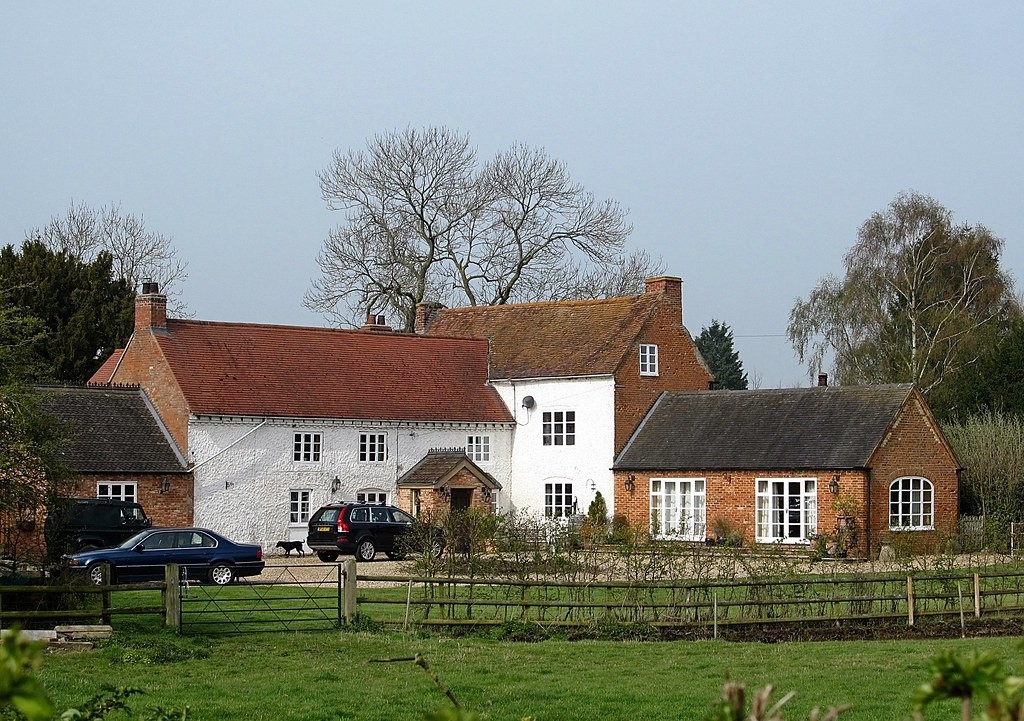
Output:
[159,476,170,493]
[625,477,634,490]
[332,476,341,492]
[829,474,840,493]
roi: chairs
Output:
[379,513,387,521]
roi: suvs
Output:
[307,502,447,561]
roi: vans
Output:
[46,496,154,560]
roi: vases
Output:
[705,538,715,546]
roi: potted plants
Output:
[479,514,505,553]
[806,496,867,554]
[709,518,742,546]
[438,512,460,552]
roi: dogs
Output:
[276,539,306,558]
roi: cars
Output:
[59,527,266,586]
